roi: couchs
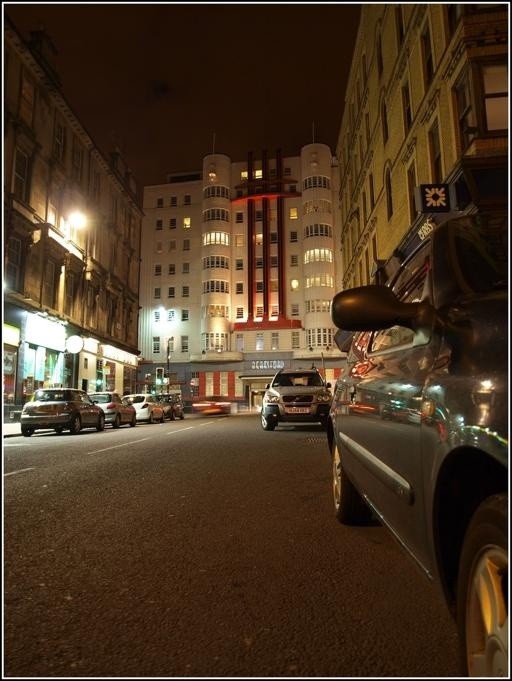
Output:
[156,368,170,386]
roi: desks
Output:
[154,321,179,397]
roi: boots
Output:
[261,367,333,431]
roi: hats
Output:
[327,209,507,677]
[20,387,185,436]
